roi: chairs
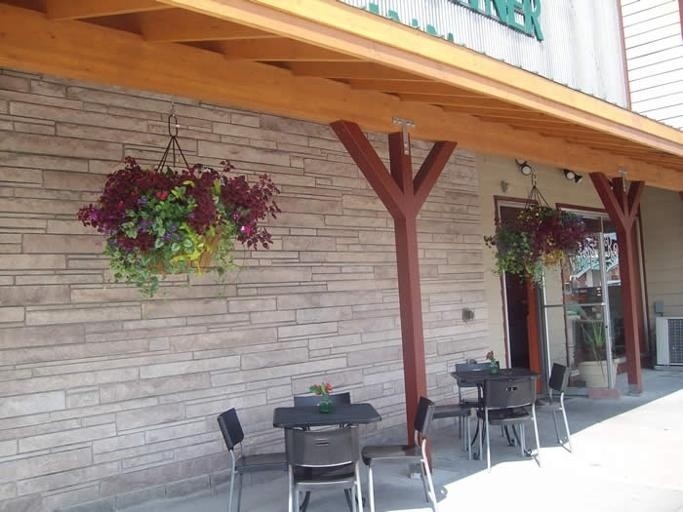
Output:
[475,374,542,471]
[362,393,440,511]
[215,407,290,510]
[281,424,364,511]
[293,390,351,429]
[455,361,505,450]
[521,363,573,453]
[431,401,474,472]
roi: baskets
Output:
[141,226,222,273]
[543,248,561,264]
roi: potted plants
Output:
[580,320,617,388]
[481,206,589,287]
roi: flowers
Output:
[486,351,498,367]
[309,380,335,394]
[75,154,282,293]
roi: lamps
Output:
[565,169,583,183]
[516,158,531,175]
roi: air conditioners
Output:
[655,316,683,366]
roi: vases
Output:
[318,393,333,414]
[490,367,498,374]
[150,230,222,275]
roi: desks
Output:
[448,369,542,460]
[272,403,383,511]
